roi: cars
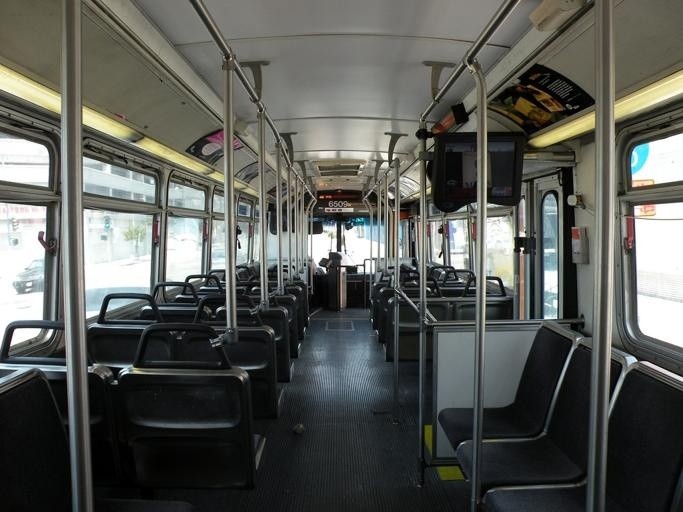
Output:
[211,236,259,263]
[10,258,44,295]
[85,274,174,319]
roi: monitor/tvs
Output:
[434,131,526,213]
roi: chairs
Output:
[0,264,310,512]
[373,266,512,418]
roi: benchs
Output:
[439,320,681,510]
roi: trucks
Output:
[463,194,558,318]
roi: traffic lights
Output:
[103,215,111,229]
[11,216,19,232]
[100,235,108,240]
[12,238,19,246]
